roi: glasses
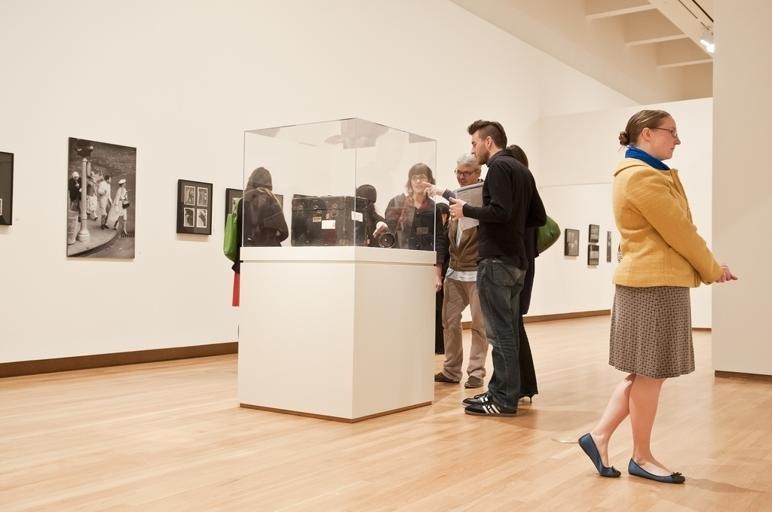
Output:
[656,127,677,137]
[453,167,477,177]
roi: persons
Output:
[232,166,289,340]
[434,152,490,387]
[357,162,449,354]
[578,108,737,484]
[449,120,546,417]
[68,170,130,237]
[425,144,546,404]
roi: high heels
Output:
[520,388,537,403]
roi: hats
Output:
[72,171,79,179]
[118,179,126,184]
[357,184,376,202]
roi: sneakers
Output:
[462,393,493,405]
[434,372,459,383]
[464,376,483,388]
[465,401,516,416]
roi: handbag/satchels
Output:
[537,216,561,254]
[122,199,130,208]
[223,200,240,261]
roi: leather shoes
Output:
[578,434,622,476]
[629,457,685,484]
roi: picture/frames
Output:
[588,245,599,265]
[293,194,317,198]
[177,180,212,235]
[225,188,283,228]
[589,224,599,242]
[0,151,14,225]
[607,231,618,262]
[565,229,579,256]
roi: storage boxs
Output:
[291,195,368,246]
